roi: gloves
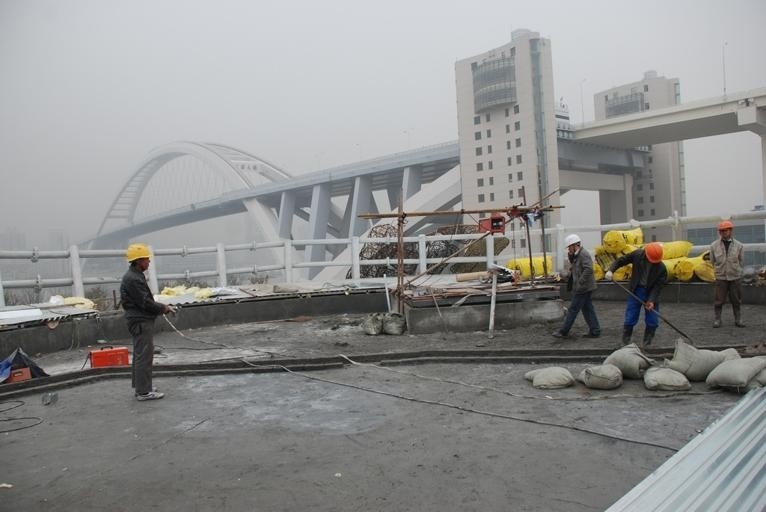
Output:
[605,271,612,281]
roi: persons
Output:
[120,243,170,402]
[710,220,748,328]
[552,234,601,339]
[605,244,667,350]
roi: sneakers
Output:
[582,332,597,338]
[135,387,157,397]
[552,331,568,339]
[137,391,164,400]
[736,320,745,327]
[713,319,721,328]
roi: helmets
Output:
[127,245,149,262]
[719,222,733,230]
[645,244,663,263]
[564,235,581,248]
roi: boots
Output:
[623,326,633,345]
[644,327,656,346]
